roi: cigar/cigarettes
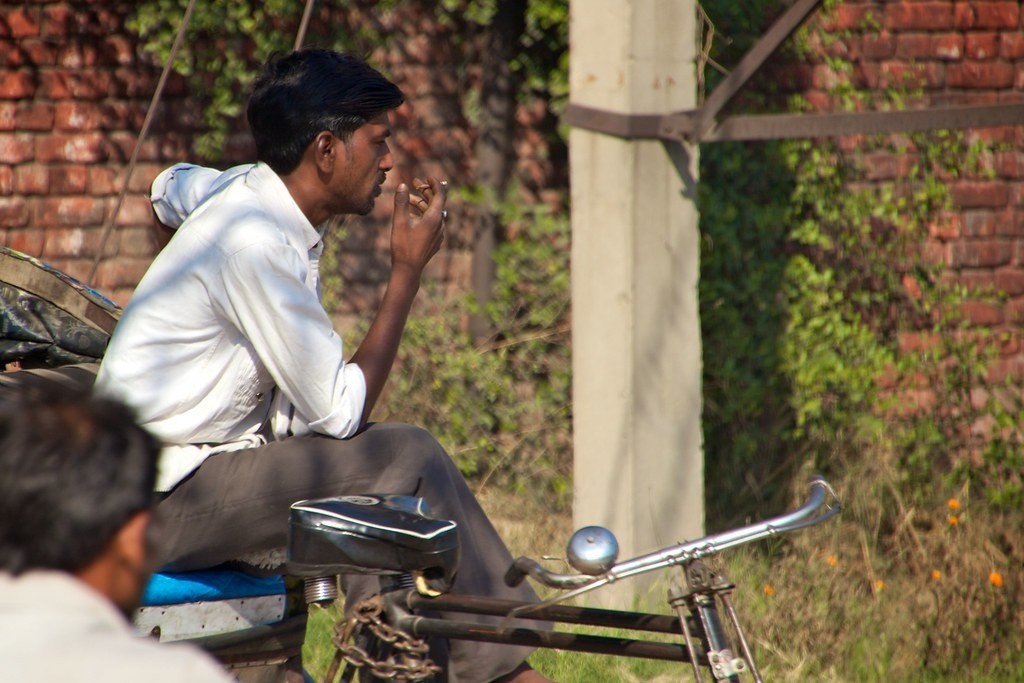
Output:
[416,181,449,190]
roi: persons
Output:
[91,44,557,683]
[1,370,237,683]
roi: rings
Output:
[441,210,448,218]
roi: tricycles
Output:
[0,246,842,683]
